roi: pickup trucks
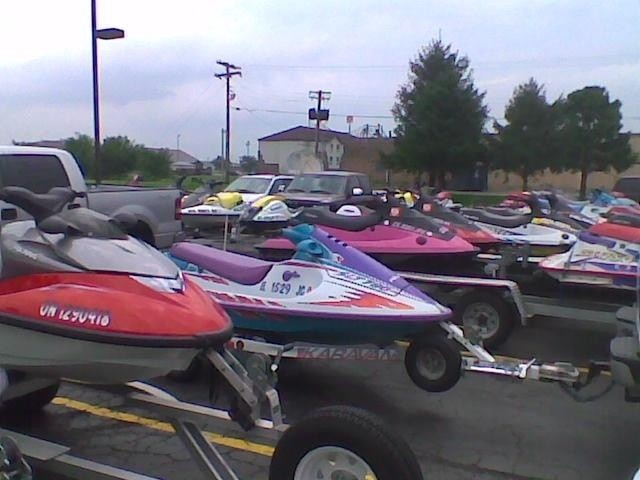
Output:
[0,145,183,251]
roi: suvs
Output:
[225,174,293,204]
[275,171,373,225]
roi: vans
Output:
[611,177,640,204]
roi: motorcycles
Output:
[179,190,254,231]
[167,174,187,191]
[235,195,304,232]
[0,179,234,386]
[165,224,453,346]
[126,173,143,186]
[181,177,229,208]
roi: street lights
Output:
[176,133,181,150]
[90,1,125,186]
